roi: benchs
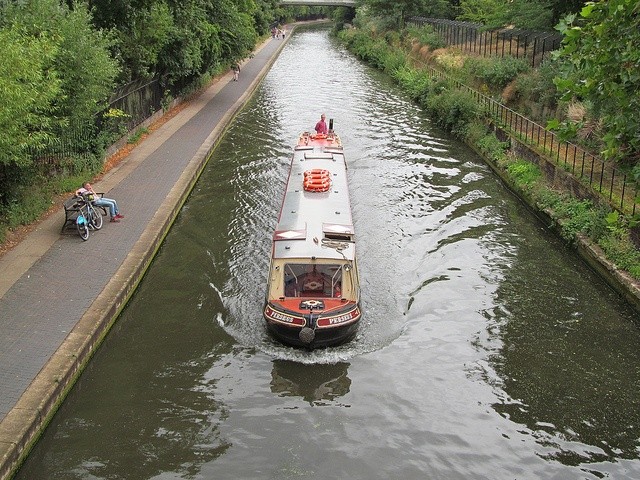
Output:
[62,191,108,234]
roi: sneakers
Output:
[116,212,125,218]
[110,216,121,223]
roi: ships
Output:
[262,127,362,353]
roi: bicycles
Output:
[74,192,103,240]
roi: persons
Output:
[233,60,240,81]
[272,27,286,39]
[75,182,124,223]
[314,114,327,134]
